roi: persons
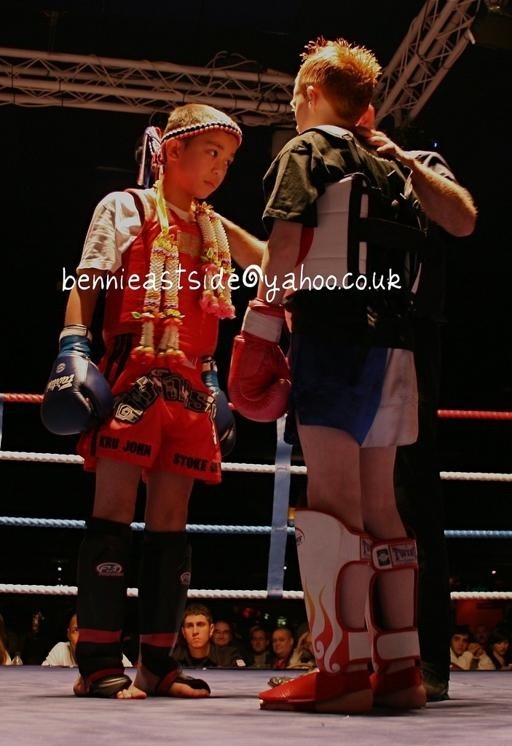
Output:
[226,42,432,714]
[41,104,240,701]
[205,104,478,701]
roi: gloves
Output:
[200,370,237,459]
[226,297,292,422]
[40,328,114,438]
